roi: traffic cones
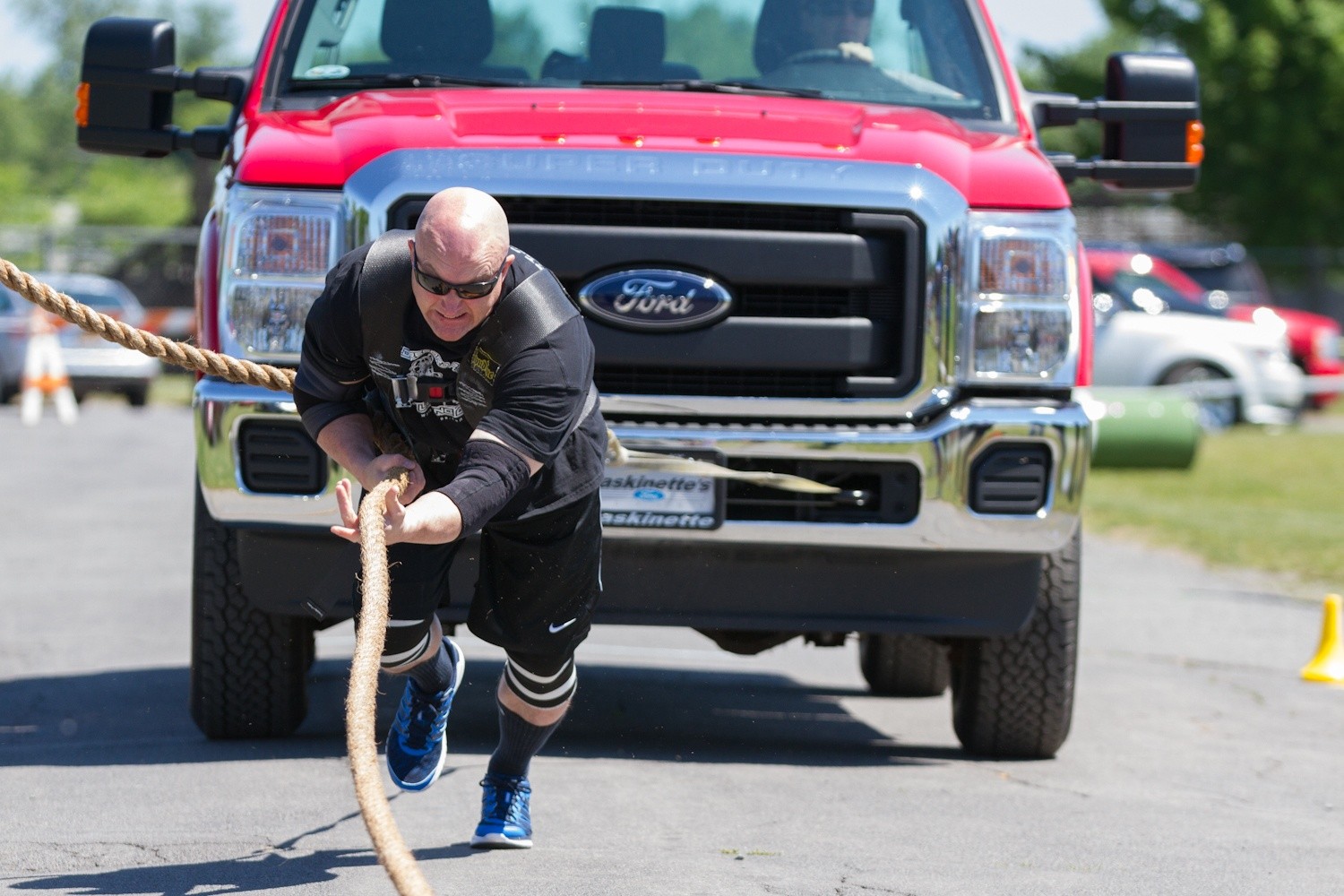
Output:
[1295,590,1344,689]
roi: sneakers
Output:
[386,636,465,792]
[470,772,535,851]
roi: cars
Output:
[1081,238,1344,433]
[0,269,159,409]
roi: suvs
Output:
[71,0,1206,765]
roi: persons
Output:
[293,187,607,848]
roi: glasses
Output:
[413,242,507,300]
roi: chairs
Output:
[344,0,532,81]
[751,0,816,78]
[540,3,703,82]
